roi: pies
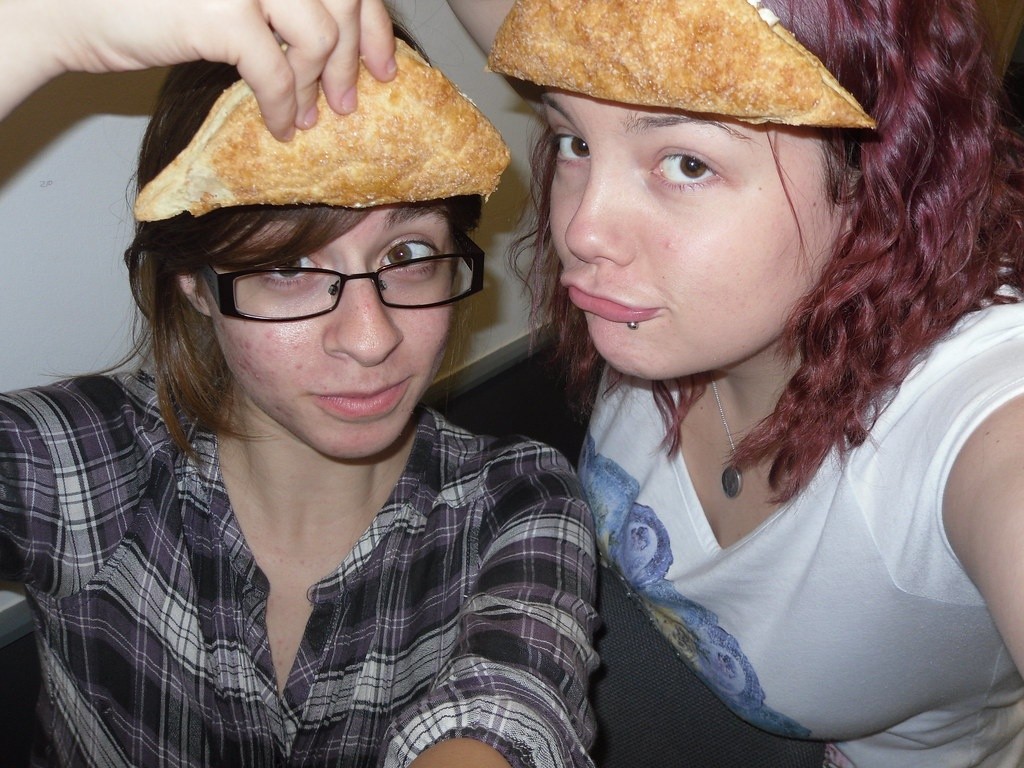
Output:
[483,0,879,129]
[131,36,512,224]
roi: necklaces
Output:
[711,375,743,499]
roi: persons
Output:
[449,0,1024,768]
[0,0,601,768]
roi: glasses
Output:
[202,231,485,322]
